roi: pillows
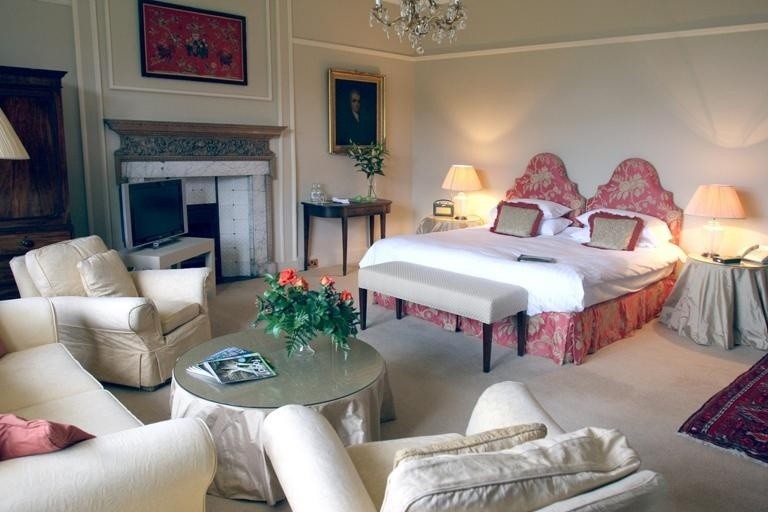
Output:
[490,197,674,252]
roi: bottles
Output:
[310,183,327,203]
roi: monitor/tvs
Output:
[120,176,188,252]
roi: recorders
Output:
[432,198,455,217]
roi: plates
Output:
[349,198,377,203]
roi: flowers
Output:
[345,138,390,198]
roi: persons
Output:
[335,89,375,145]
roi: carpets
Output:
[676,350,768,466]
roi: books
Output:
[332,195,361,204]
[185,345,280,384]
[516,254,558,264]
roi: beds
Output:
[359,153,683,366]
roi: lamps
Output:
[368,0,471,54]
[440,165,481,220]
[683,184,745,259]
[0,109,32,162]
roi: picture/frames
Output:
[329,68,386,156]
[138,0,247,86]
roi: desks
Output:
[301,198,392,276]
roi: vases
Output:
[366,176,376,199]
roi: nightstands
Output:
[659,254,768,351]
[416,214,486,234]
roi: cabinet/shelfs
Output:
[0,65,74,300]
